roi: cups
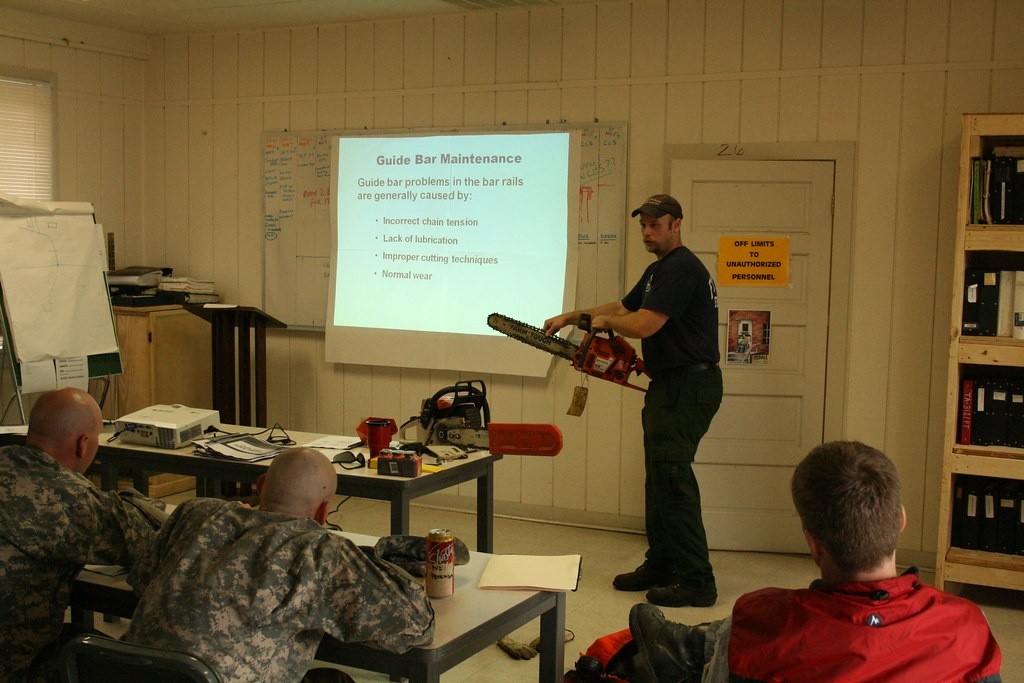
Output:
[366,419,392,459]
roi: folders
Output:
[106,265,174,289]
[962,262,1024,339]
[959,372,1024,446]
[950,475,1024,555]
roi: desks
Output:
[1,425,505,554]
[67,503,567,683]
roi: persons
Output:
[627,440,1005,683]
[0,386,166,683]
[114,446,438,683]
[540,192,723,608]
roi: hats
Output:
[631,193,683,220]
[372,535,469,578]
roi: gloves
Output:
[497,636,538,661]
[529,628,575,653]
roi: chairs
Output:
[74,632,224,682]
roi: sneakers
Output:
[645,582,718,608]
[613,562,677,591]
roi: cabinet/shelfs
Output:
[931,110,1023,596]
[89,305,215,499]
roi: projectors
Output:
[115,404,220,449]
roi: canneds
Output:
[425,529,454,598]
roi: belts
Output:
[652,361,718,382]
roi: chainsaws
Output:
[398,379,564,456]
[486,310,656,393]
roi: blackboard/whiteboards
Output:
[257,121,630,350]
[0,199,128,394]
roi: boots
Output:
[629,603,720,683]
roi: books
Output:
[966,152,1024,226]
[477,552,583,592]
